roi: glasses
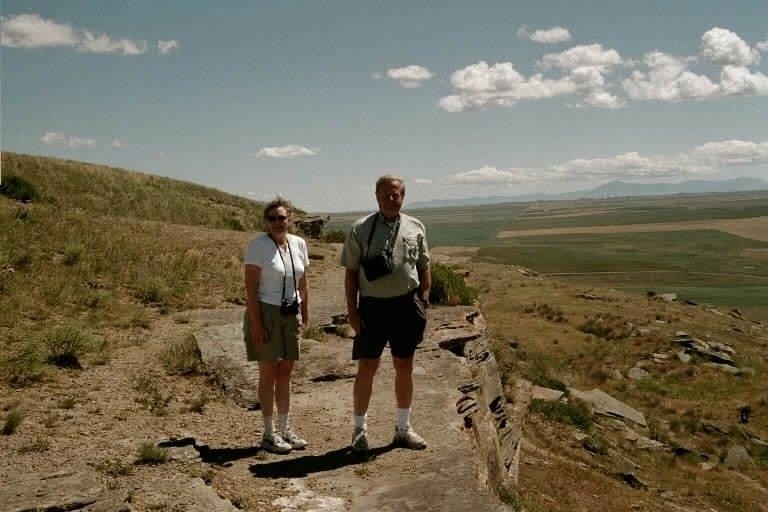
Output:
[267,216,285,221]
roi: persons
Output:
[339,175,432,454]
[242,197,310,453]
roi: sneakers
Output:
[351,429,368,452]
[260,431,307,453]
[392,425,426,449]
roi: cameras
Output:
[280,298,299,317]
